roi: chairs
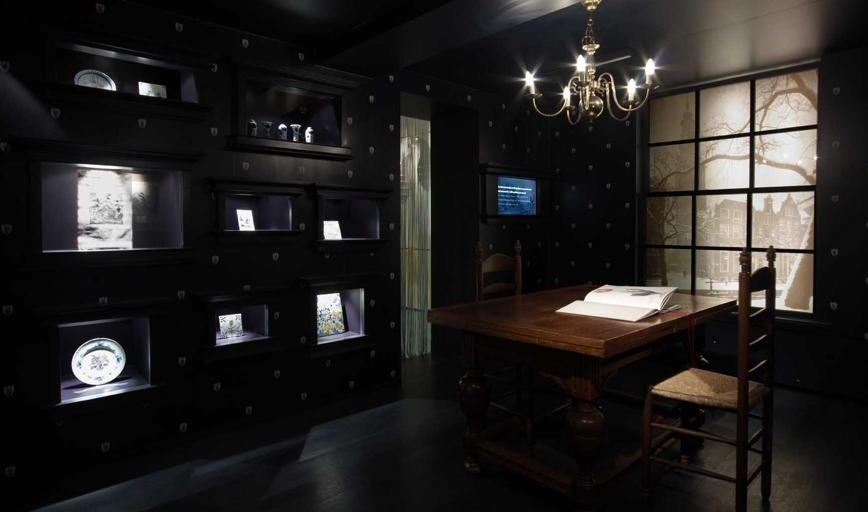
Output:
[642,242,777,512]
[470,237,581,446]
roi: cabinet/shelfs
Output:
[30,32,395,426]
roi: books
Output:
[555,284,682,323]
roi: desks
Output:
[426,283,738,512]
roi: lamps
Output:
[515,0,666,127]
